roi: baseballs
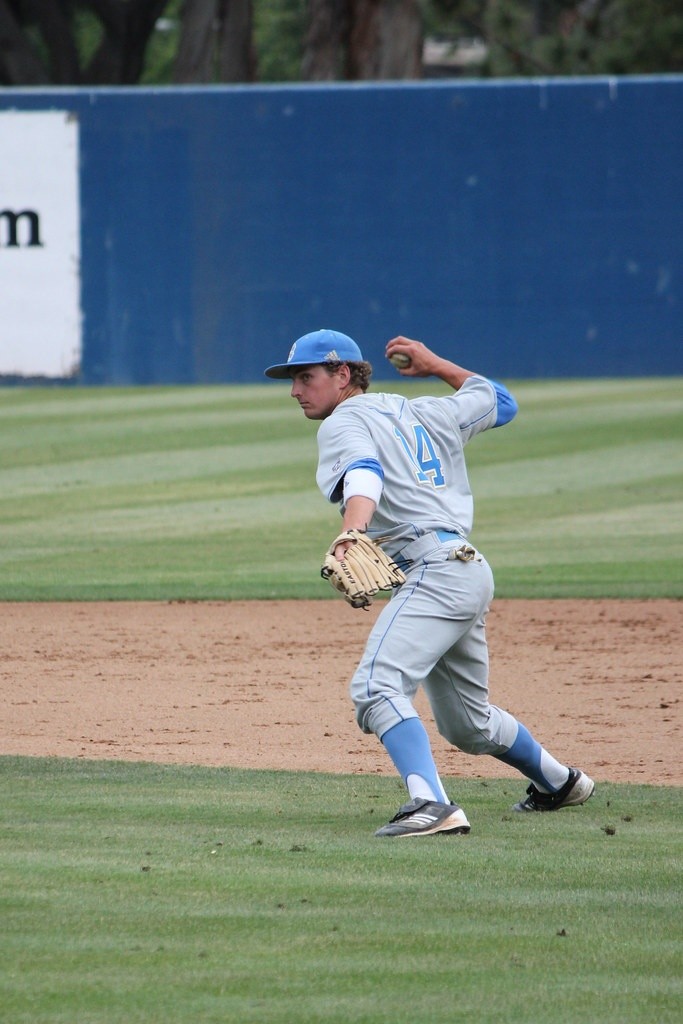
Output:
[388,353,410,369]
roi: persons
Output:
[263,330,593,837]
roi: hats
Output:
[265,329,363,380]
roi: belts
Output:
[393,531,460,572]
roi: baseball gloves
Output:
[319,522,415,612]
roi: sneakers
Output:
[373,797,470,839]
[514,768,594,812]
